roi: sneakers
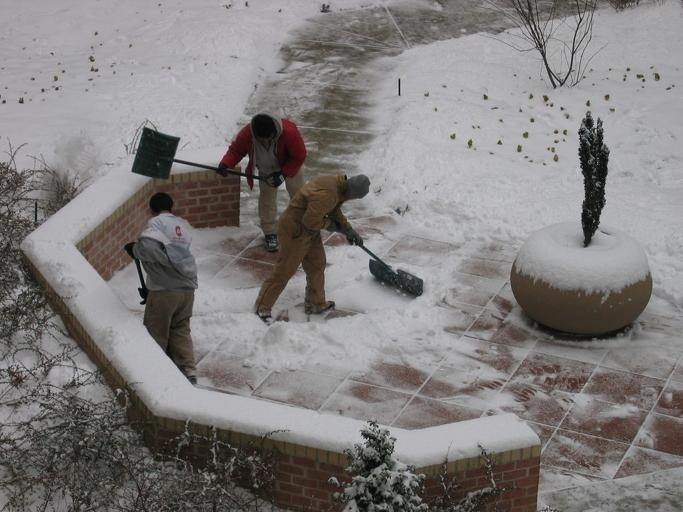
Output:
[305,300,336,315]
[264,233,280,252]
[254,312,275,325]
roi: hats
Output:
[252,114,278,136]
[348,175,370,199]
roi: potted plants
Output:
[509,110,652,336]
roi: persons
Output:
[132,190,198,386]
[216,111,310,252]
[252,171,372,325]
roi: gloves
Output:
[215,162,228,176]
[336,222,363,246]
[266,171,286,188]
